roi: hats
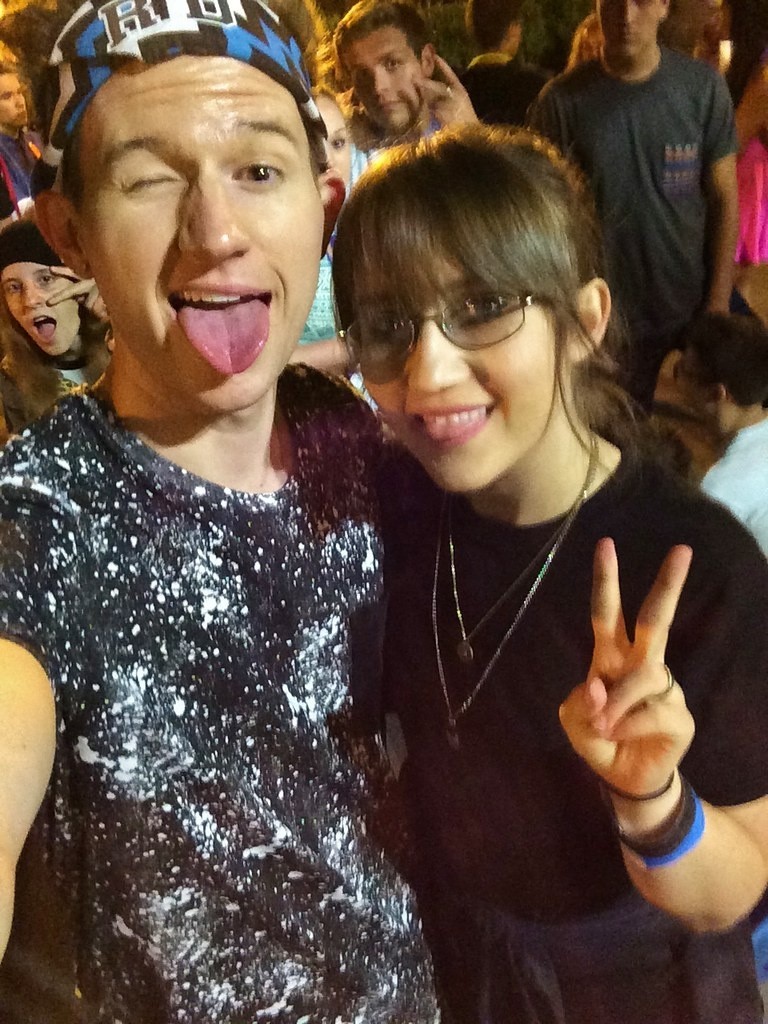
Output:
[0,220,66,272]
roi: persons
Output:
[0,0,448,1024]
[329,126,768,1024]
[288,0,607,412]
[0,219,115,451]
[692,1,768,303]
[531,0,768,419]
[677,312,768,556]
[0,0,93,234]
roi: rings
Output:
[664,664,674,692]
[446,84,450,95]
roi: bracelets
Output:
[594,770,674,801]
[599,772,705,867]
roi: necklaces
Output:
[432,424,599,728]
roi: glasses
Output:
[337,288,540,384]
